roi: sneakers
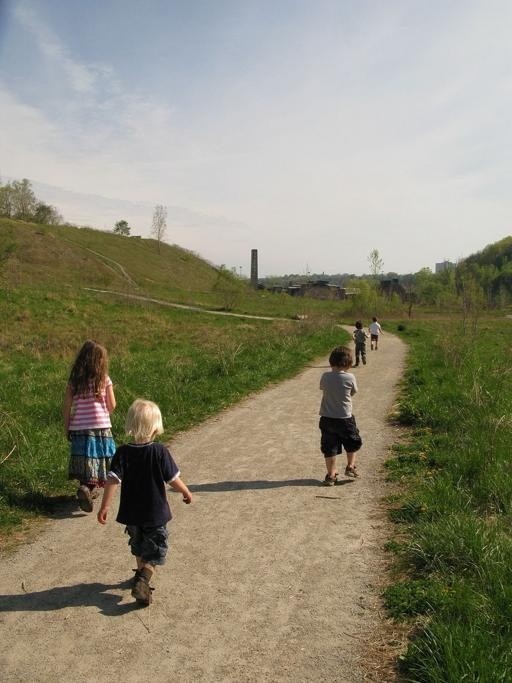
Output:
[77,485,99,512]
[345,465,362,479]
[132,568,155,605]
[324,473,339,485]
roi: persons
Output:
[62,337,118,514]
[95,396,195,607]
[367,315,385,351]
[317,344,363,486]
[351,320,370,367]
